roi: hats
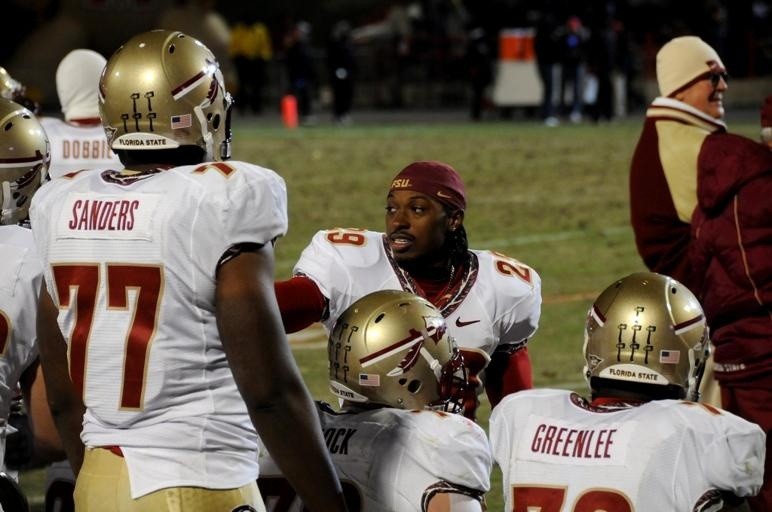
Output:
[655,35,728,98]
[389,160,466,213]
[55,48,107,121]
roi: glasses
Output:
[697,71,727,85]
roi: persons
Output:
[269,160,542,512]
[0,27,364,512]
[628,37,772,512]
[0,0,772,127]
[488,272,772,512]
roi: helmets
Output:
[582,271,710,399]
[0,97,51,225]
[0,66,16,97]
[97,29,231,163]
[327,289,455,411]
